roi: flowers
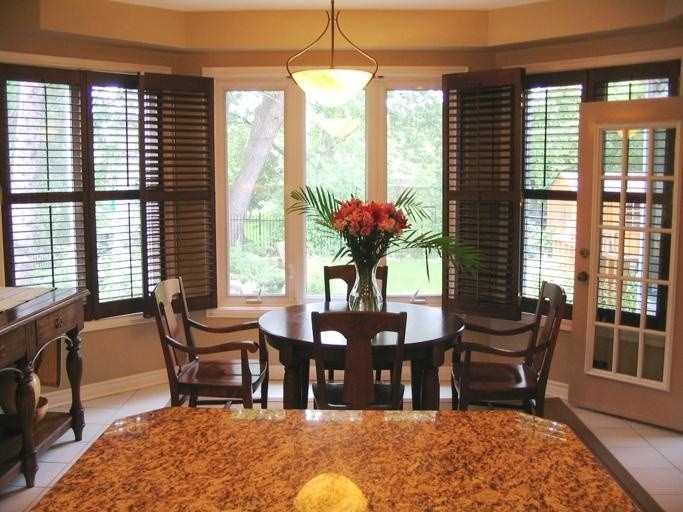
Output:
[282,184,491,311]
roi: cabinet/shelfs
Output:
[0,286,92,494]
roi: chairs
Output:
[449,278,568,418]
[150,275,270,409]
[308,311,407,411]
[323,264,389,385]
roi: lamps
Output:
[285,1,380,110]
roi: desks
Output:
[258,300,466,410]
